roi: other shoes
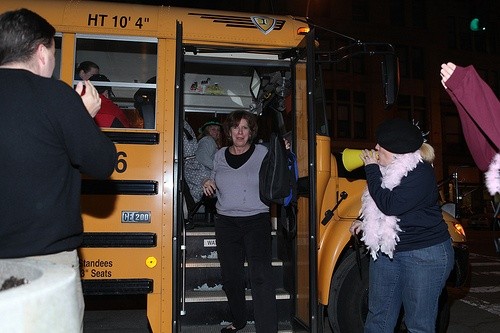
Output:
[221,323,247,333]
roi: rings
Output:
[205,186,208,189]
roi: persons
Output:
[74,61,157,129]
[196,117,225,215]
[440,62,500,197]
[202,109,294,333]
[0,8,118,333]
[349,116,454,333]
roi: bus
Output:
[0,0,470,333]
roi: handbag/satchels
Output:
[258,132,298,208]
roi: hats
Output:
[376,118,422,155]
[203,116,224,129]
